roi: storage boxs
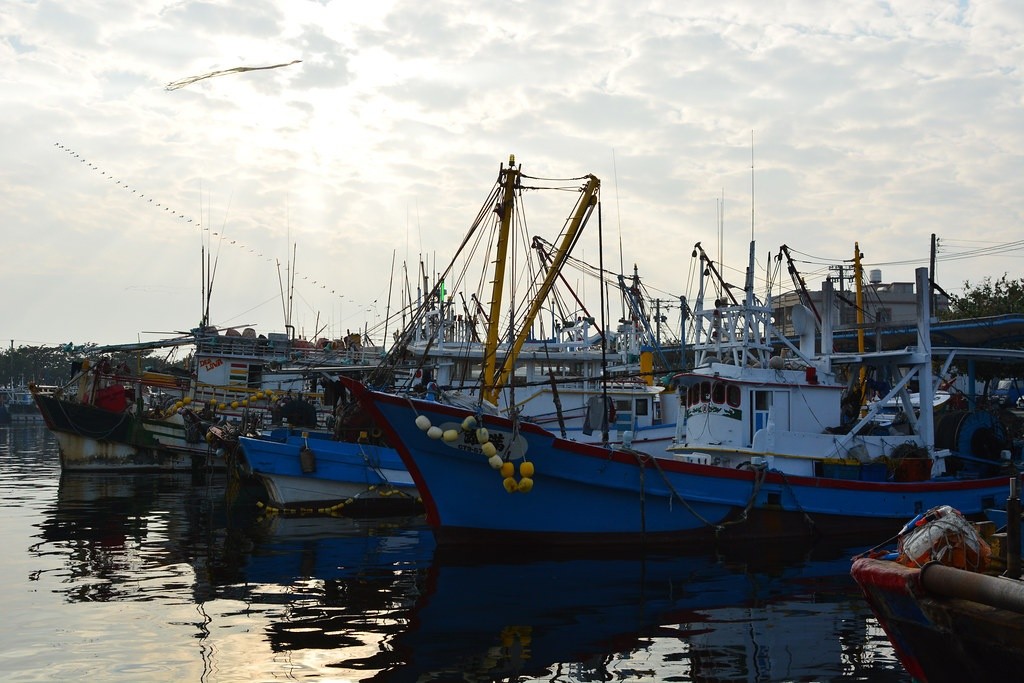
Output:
[814,457,933,482]
[94,385,126,412]
[900,532,969,567]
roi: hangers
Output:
[596,392,603,397]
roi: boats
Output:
[326,542,894,683]
[1,156,1024,552]
[851,478,1024,683]
